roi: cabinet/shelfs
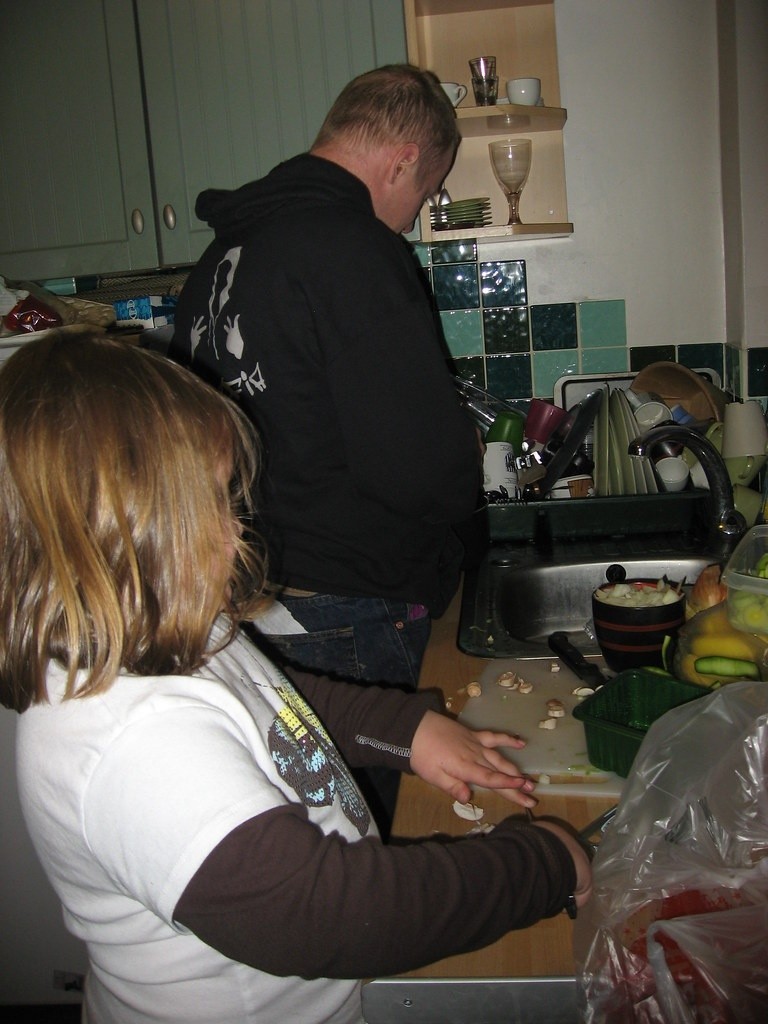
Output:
[404,0,574,246]
[0,0,406,281]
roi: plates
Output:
[539,383,659,499]
[430,198,493,232]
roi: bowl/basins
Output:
[630,361,730,422]
[634,402,673,434]
[682,447,710,490]
[719,525,768,635]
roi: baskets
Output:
[571,668,715,779]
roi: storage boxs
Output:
[570,669,717,778]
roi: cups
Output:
[525,398,567,445]
[706,400,768,528]
[486,412,524,457]
[508,78,542,106]
[591,579,686,674]
[482,441,522,503]
[656,458,689,491]
[438,56,498,108]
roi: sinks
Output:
[456,533,714,661]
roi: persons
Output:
[0,331,590,1024]
[174,63,485,855]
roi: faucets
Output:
[628,425,747,559]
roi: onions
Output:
[594,576,685,607]
[690,564,727,610]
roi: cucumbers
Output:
[694,656,761,681]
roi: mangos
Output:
[678,611,768,688]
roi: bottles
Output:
[670,404,696,425]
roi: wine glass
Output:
[488,138,533,225]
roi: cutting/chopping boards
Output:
[455,656,622,797]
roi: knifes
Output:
[549,633,607,691]
[524,806,577,920]
